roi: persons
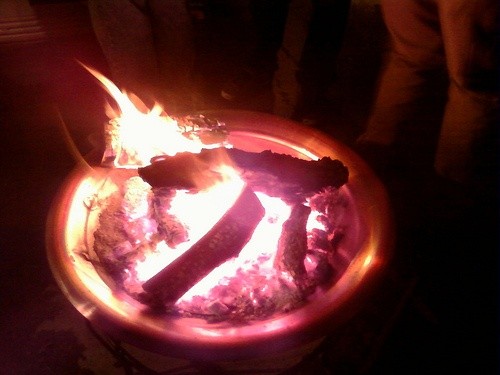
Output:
[236,1,351,126]
[28,0,210,142]
[349,0,499,205]
